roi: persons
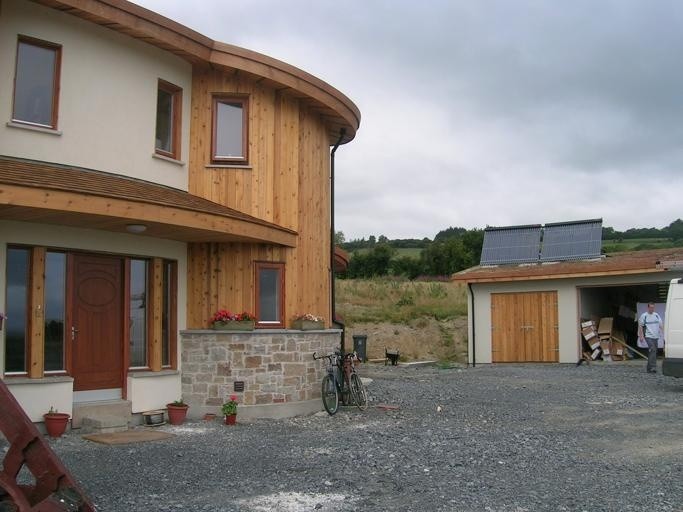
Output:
[636,301,664,374]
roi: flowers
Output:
[292,311,325,322]
[229,395,236,404]
[210,309,256,323]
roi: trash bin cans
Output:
[353,335,367,363]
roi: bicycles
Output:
[311,346,368,414]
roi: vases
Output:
[203,413,215,420]
[293,322,324,331]
[212,320,255,331]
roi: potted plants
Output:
[166,397,188,426]
[222,401,238,425]
[42,404,69,436]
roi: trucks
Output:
[659,277,682,378]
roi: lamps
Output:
[126,224,147,234]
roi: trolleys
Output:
[383,347,400,366]
